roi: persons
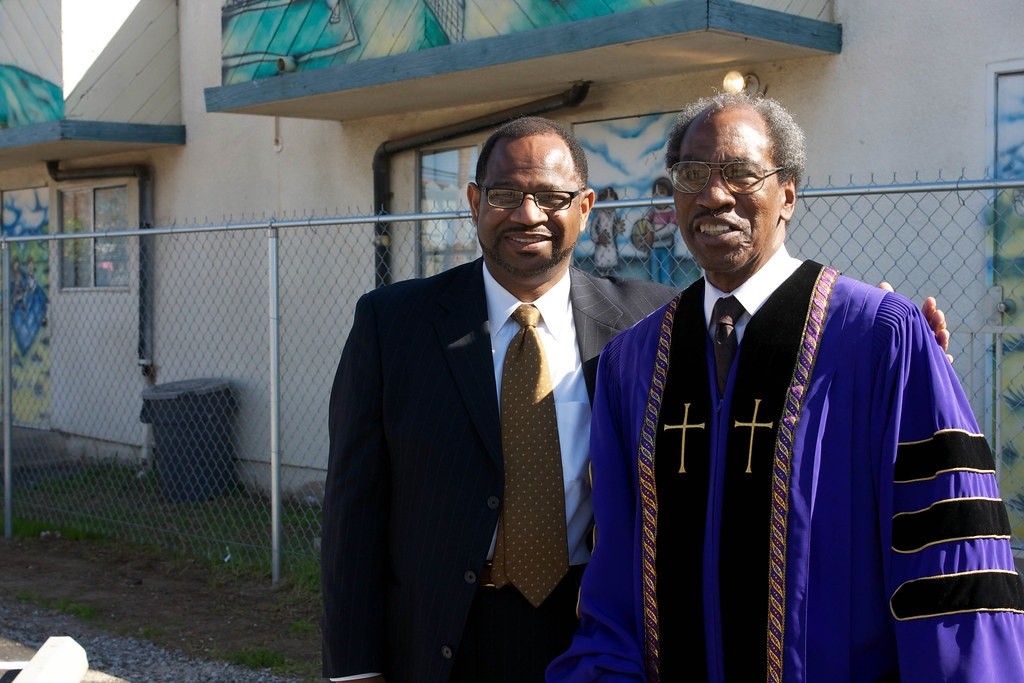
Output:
[317,113,955,683]
[548,87,1024,683]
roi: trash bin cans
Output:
[140,376,240,508]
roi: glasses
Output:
[476,183,587,211]
[667,161,792,194]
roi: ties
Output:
[490,304,569,608]
[712,294,747,406]
